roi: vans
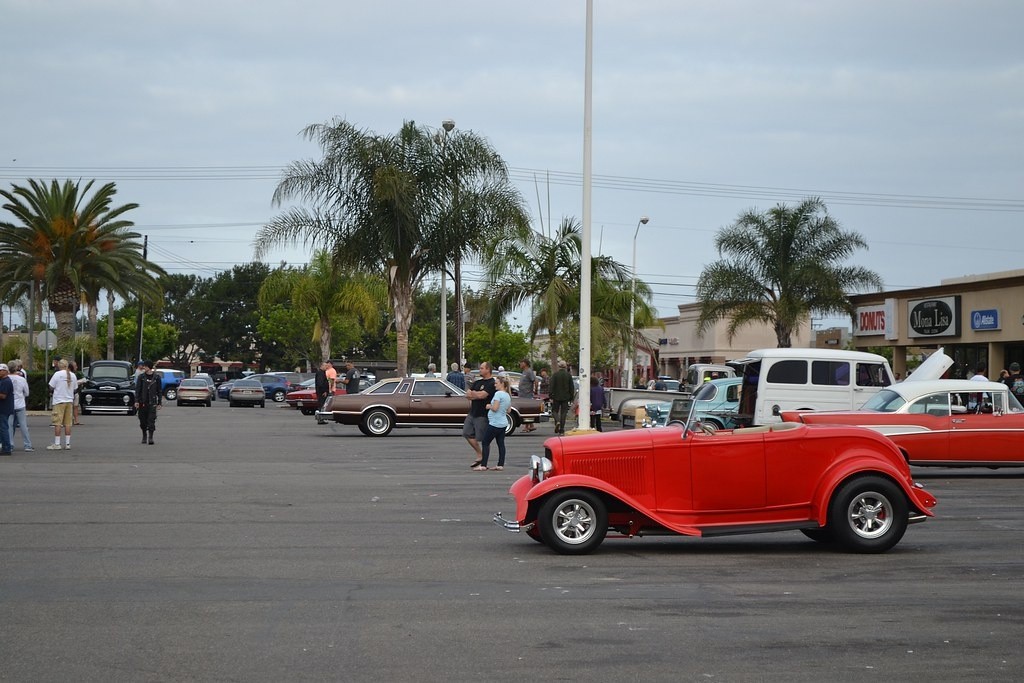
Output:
[150,369,184,400]
[726,346,954,425]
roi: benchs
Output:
[733,422,803,435]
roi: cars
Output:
[313,378,550,438]
[176,379,211,407]
[654,376,685,392]
[80,360,137,416]
[410,369,543,391]
[494,396,938,553]
[229,379,266,408]
[283,372,377,415]
[642,377,744,431]
[217,372,303,402]
[780,378,1024,468]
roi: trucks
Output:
[604,363,737,422]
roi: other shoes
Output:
[74,422,84,425]
[473,464,488,470]
[560,433,564,435]
[489,464,503,470]
[0,450,12,456]
[149,435,154,444]
[317,421,328,425]
[142,434,148,443]
[555,422,561,434]
[10,445,14,451]
[25,448,35,451]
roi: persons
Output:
[590,376,607,432]
[952,361,1024,407]
[473,375,511,471]
[549,360,574,436]
[498,366,505,375]
[446,362,465,390]
[134,360,146,383]
[518,359,538,433]
[325,359,337,395]
[0,356,83,456]
[540,368,550,411]
[335,361,360,394]
[315,362,329,424]
[463,361,498,467]
[134,359,162,444]
[895,373,903,383]
[424,363,436,377]
[464,362,476,392]
[636,376,688,392]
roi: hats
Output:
[463,363,472,369]
[143,359,154,369]
[498,366,504,371]
[137,360,144,365]
[0,363,9,371]
[326,360,333,364]
[53,356,61,361]
[557,360,566,368]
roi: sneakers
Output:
[47,444,62,449]
[64,444,71,450]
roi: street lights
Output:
[441,118,456,381]
[626,216,649,390]
[10,279,34,374]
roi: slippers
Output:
[520,427,537,432]
[470,458,483,467]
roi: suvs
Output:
[193,373,216,400]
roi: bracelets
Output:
[535,392,537,394]
